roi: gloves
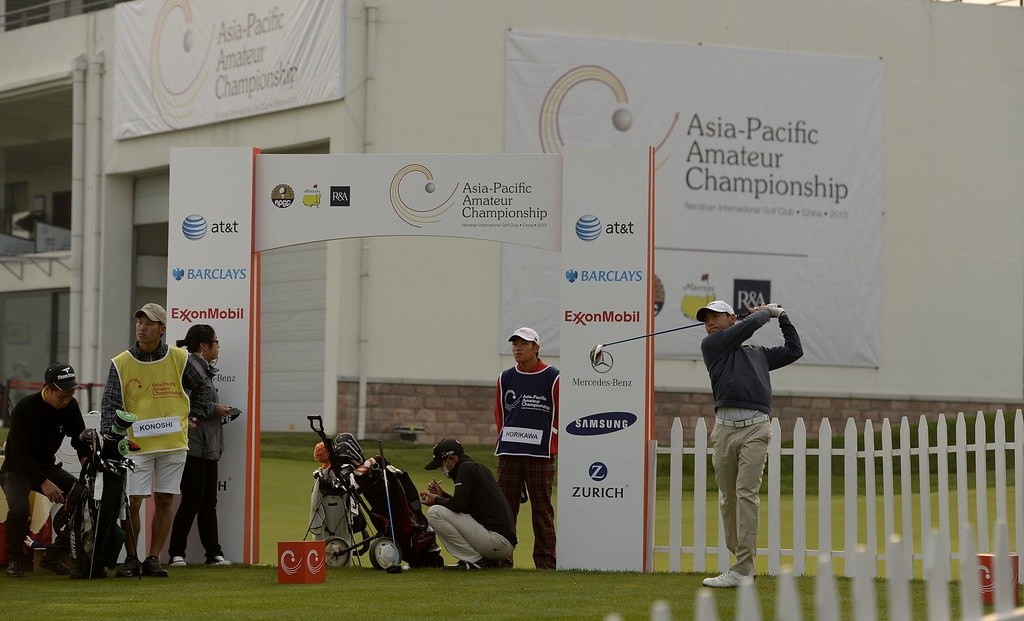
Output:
[749,302,786,318]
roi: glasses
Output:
[210,340,218,345]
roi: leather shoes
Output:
[702,565,756,588]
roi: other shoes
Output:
[70,565,109,578]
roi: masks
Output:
[441,459,454,479]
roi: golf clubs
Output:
[378,439,403,574]
[589,304,783,365]
[107,457,135,475]
[312,463,355,490]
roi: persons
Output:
[166,325,230,567]
[99,303,213,577]
[418,438,517,572]
[697,300,803,588]
[0,361,92,578]
[495,327,560,570]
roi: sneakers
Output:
[6,560,26,578]
[455,559,489,570]
[40,552,71,574]
[168,556,187,567]
[142,556,169,577]
[116,555,143,578]
[494,557,513,568]
[204,555,231,567]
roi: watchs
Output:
[189,416,200,424]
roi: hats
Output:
[44,361,82,391]
[134,303,167,327]
[507,327,540,347]
[696,301,735,322]
[424,438,464,470]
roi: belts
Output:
[714,414,769,429]
